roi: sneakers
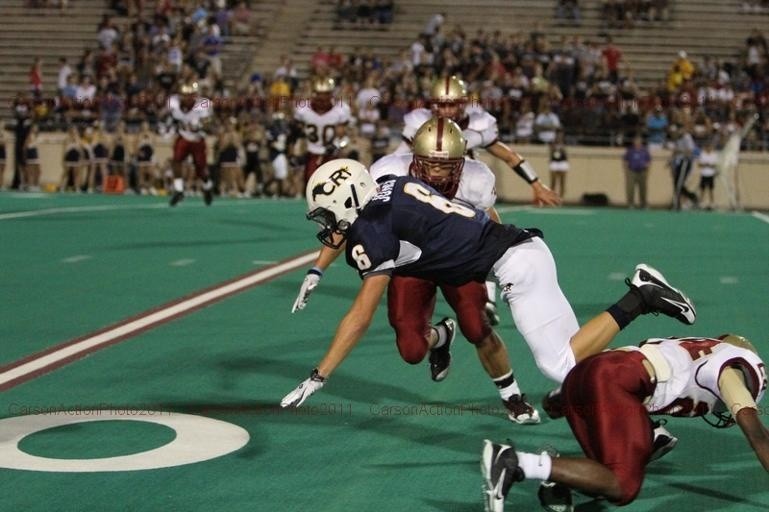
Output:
[169,188,212,206]
[502,393,540,424]
[624,263,696,325]
[429,317,456,381]
[484,301,499,325]
[480,439,574,512]
[646,419,678,462]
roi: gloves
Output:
[291,268,321,313]
[280,374,324,408]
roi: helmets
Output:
[701,333,758,428]
[306,158,379,249]
[409,75,469,199]
[312,76,335,93]
[179,82,199,96]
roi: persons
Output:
[1,0,769,218]
[277,156,695,468]
[290,116,541,424]
[477,333,769,511]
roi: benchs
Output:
[1,1,769,150]
